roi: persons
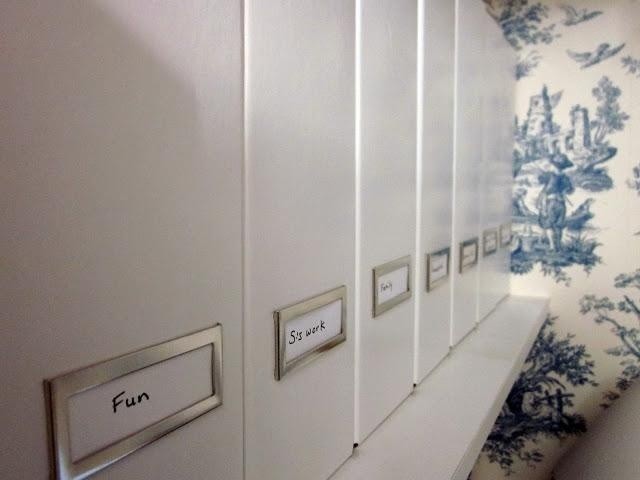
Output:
[532,150,578,257]
